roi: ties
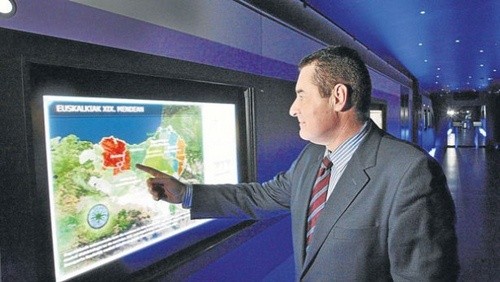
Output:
[305,156,332,252]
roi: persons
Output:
[135,45,459,282]
